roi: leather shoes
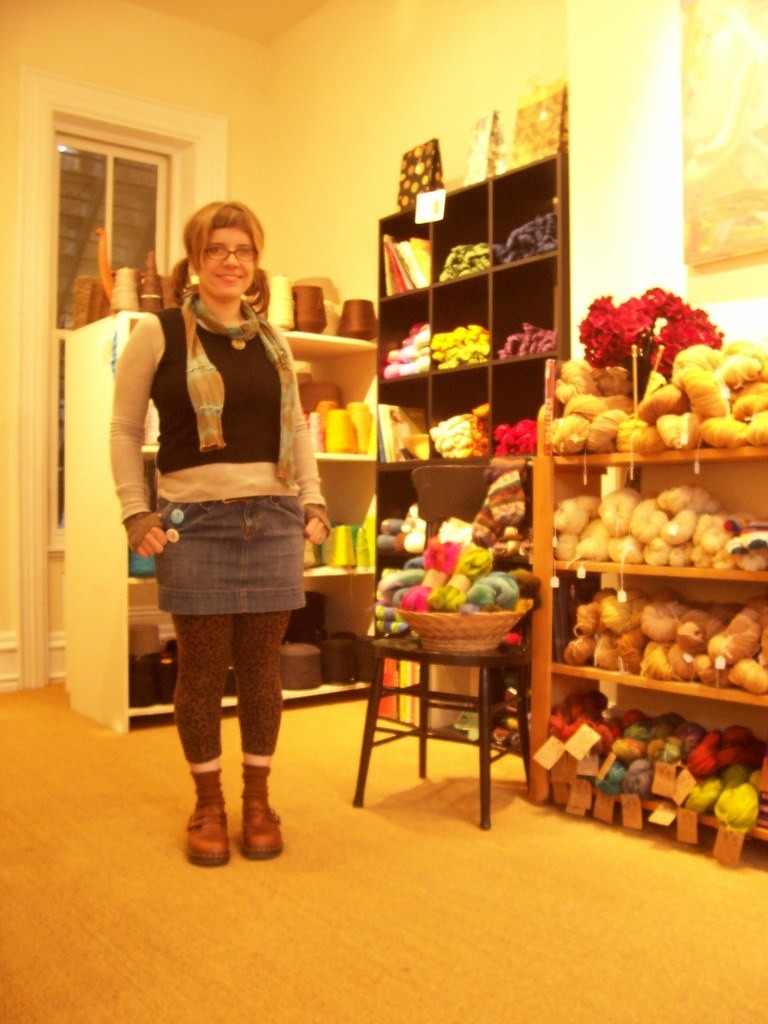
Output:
[186,804,229,866]
[242,800,284,860]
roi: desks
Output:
[353,636,532,831]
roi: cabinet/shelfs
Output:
[64,309,377,734]
[373,151,569,656]
[530,355,768,841]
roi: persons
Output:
[109,201,331,866]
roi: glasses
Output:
[204,247,258,261]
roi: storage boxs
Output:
[378,657,480,728]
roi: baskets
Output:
[397,599,535,654]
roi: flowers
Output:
[577,287,726,376]
